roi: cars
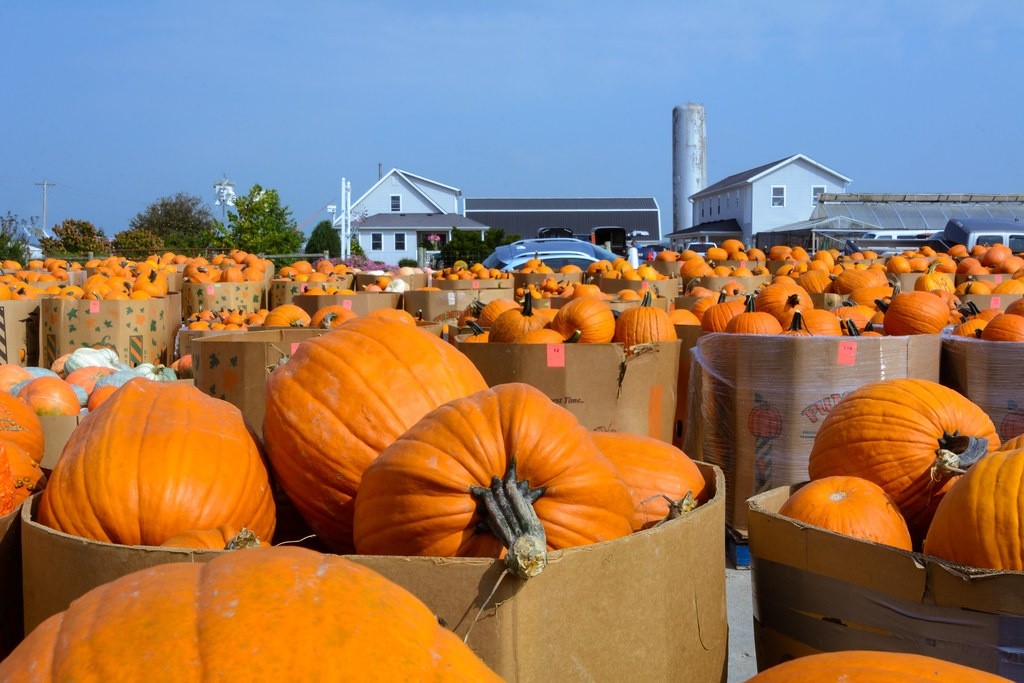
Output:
[634,242,668,268]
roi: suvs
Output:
[685,241,718,257]
[481,237,626,274]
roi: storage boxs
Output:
[0,261,1024,683]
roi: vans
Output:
[858,230,945,258]
[967,229,1024,255]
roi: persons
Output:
[626,240,639,270]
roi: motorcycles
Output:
[535,227,574,238]
[588,226,627,257]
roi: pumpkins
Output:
[0,239,1024,683]
